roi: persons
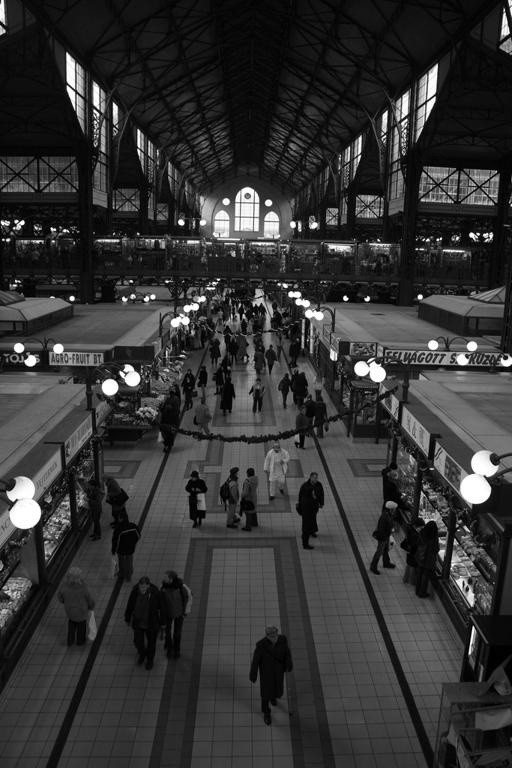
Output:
[157,569,194,661]
[156,283,328,450]
[81,478,106,542]
[56,565,97,648]
[109,509,141,584]
[103,476,130,529]
[185,470,208,528]
[247,624,293,727]
[241,468,258,531]
[226,467,240,528]
[123,575,168,671]
[368,460,440,598]
[264,441,290,501]
[299,472,325,551]
